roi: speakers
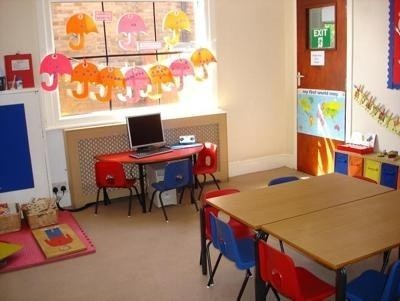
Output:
[179,136,195,144]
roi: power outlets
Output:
[51,180,68,195]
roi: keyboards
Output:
[129,148,173,159]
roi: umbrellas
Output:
[39,8,217,104]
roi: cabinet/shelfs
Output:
[333,147,400,193]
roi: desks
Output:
[93,140,206,214]
[198,172,395,301]
[250,189,400,300]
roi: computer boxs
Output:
[145,163,178,208]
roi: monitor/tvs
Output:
[126,113,166,149]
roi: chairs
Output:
[198,188,256,289]
[148,157,199,223]
[202,210,281,301]
[351,175,377,187]
[185,140,221,202]
[343,259,400,301]
[267,175,302,255]
[255,237,337,300]
[94,158,145,219]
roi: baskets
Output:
[0,203,22,235]
[21,203,59,231]
